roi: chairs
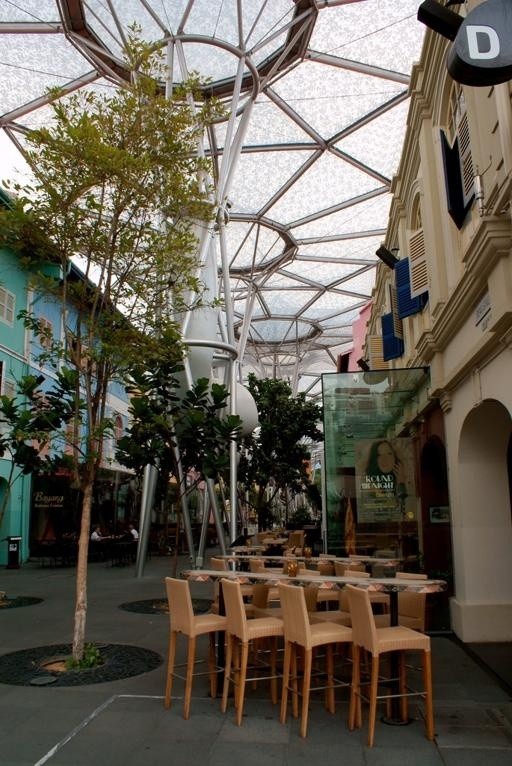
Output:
[37,536,154,570]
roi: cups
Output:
[304,547,312,559]
[288,560,298,577]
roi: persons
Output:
[90,524,108,541]
[365,440,408,499]
[128,523,138,538]
[118,528,134,563]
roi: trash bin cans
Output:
[372,565,396,579]
[8,536,21,569]
[375,550,395,558]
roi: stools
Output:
[345,583,438,747]
[219,577,284,728]
[277,582,364,738]
[207,525,432,692]
[164,575,226,721]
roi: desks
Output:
[182,567,446,727]
